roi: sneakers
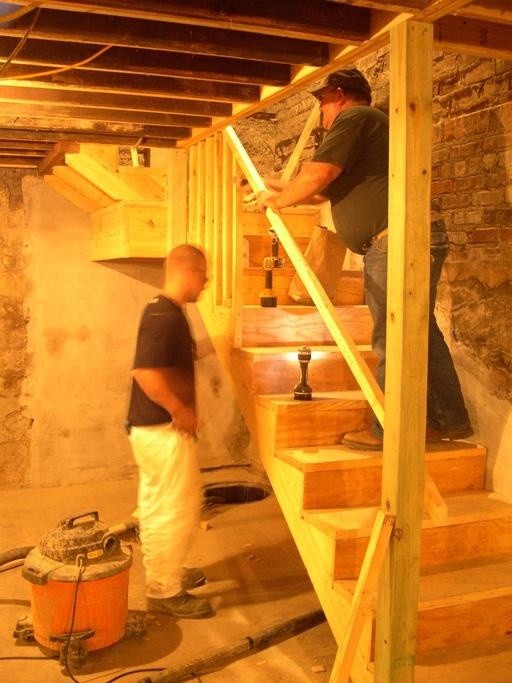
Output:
[426,420,474,443]
[341,420,384,451]
[147,566,213,618]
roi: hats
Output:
[310,68,371,98]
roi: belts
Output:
[362,209,443,252]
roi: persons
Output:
[122,242,214,619]
[235,64,476,451]
